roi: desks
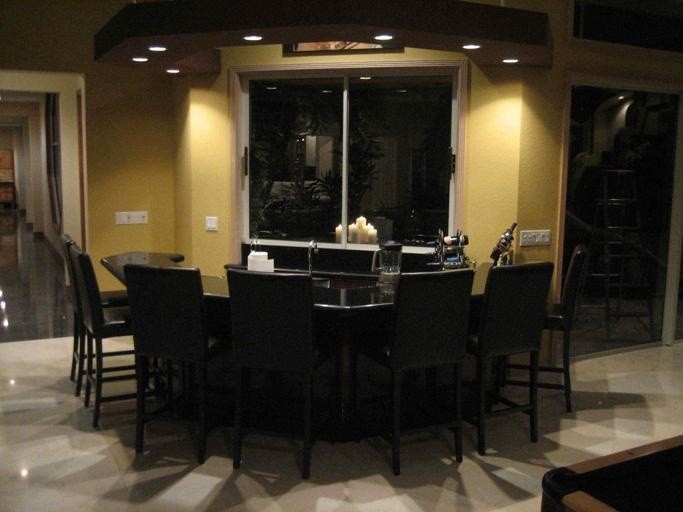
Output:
[541,434,682,512]
[98,252,498,444]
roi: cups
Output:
[370,242,403,276]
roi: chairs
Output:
[486,243,587,416]
[123,261,227,465]
[339,267,475,478]
[62,237,131,397]
[223,266,325,480]
[67,244,134,431]
[460,260,555,456]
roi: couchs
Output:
[261,181,333,234]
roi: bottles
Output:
[490,223,518,260]
[426,234,469,268]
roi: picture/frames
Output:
[281,37,405,57]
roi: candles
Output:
[335,213,378,244]
